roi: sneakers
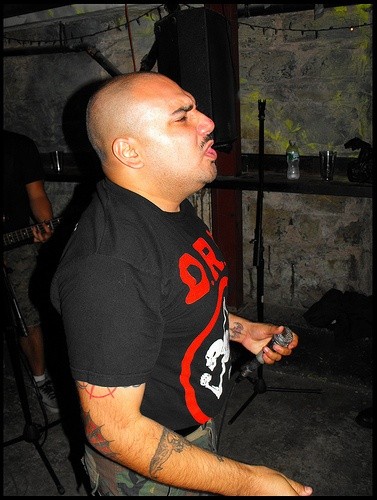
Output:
[32,369,61,414]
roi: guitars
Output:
[0,216,79,253]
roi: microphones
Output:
[234,325,293,384]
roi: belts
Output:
[174,425,200,437]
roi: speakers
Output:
[153,7,236,148]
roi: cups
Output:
[319,148,337,182]
[47,137,64,171]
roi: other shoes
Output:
[355,406,373,429]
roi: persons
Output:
[0,128,64,415]
[51,73,314,497]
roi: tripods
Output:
[227,99,321,427]
[0,257,65,494]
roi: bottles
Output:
[286,140,300,179]
